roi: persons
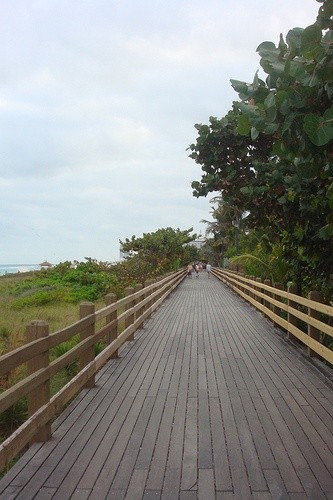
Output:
[182,258,215,281]
